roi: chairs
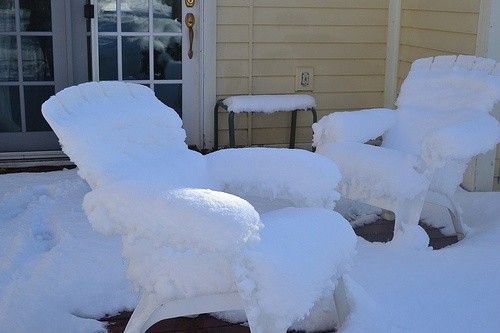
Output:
[39,80,352,333]
[316,53,500,249]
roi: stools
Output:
[212,97,320,153]
